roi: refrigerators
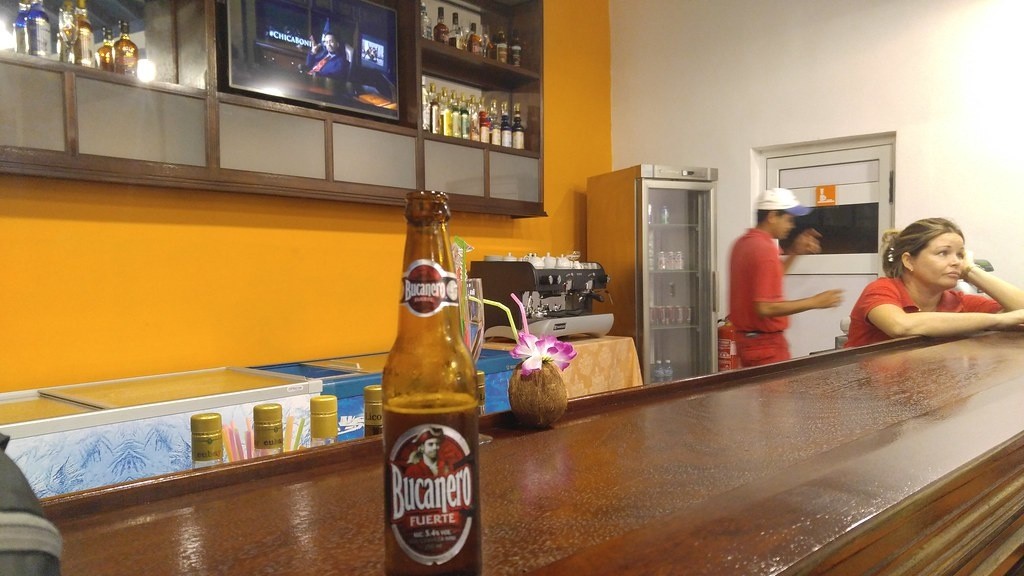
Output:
[586,163,719,386]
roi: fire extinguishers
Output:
[718,315,740,373]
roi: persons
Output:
[841,217,1024,350]
[299,31,350,81]
[727,187,846,368]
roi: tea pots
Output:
[485,252,585,270]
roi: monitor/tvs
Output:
[215,0,400,124]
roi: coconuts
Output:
[507,359,568,429]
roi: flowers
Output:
[508,333,578,375]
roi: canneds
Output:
[659,205,670,224]
[657,251,685,269]
[648,203,652,223]
[649,304,694,325]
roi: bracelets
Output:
[962,262,986,283]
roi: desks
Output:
[479,336,645,401]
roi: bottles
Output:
[477,370,486,416]
[364,384,382,437]
[653,359,664,383]
[421,76,525,150]
[13,0,138,76]
[421,2,522,66]
[656,250,684,270]
[310,394,338,448]
[664,358,673,382]
[253,403,283,457]
[659,205,669,224]
[381,188,482,576]
[191,413,224,469]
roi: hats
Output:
[757,189,812,216]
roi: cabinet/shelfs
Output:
[400,0,545,158]
